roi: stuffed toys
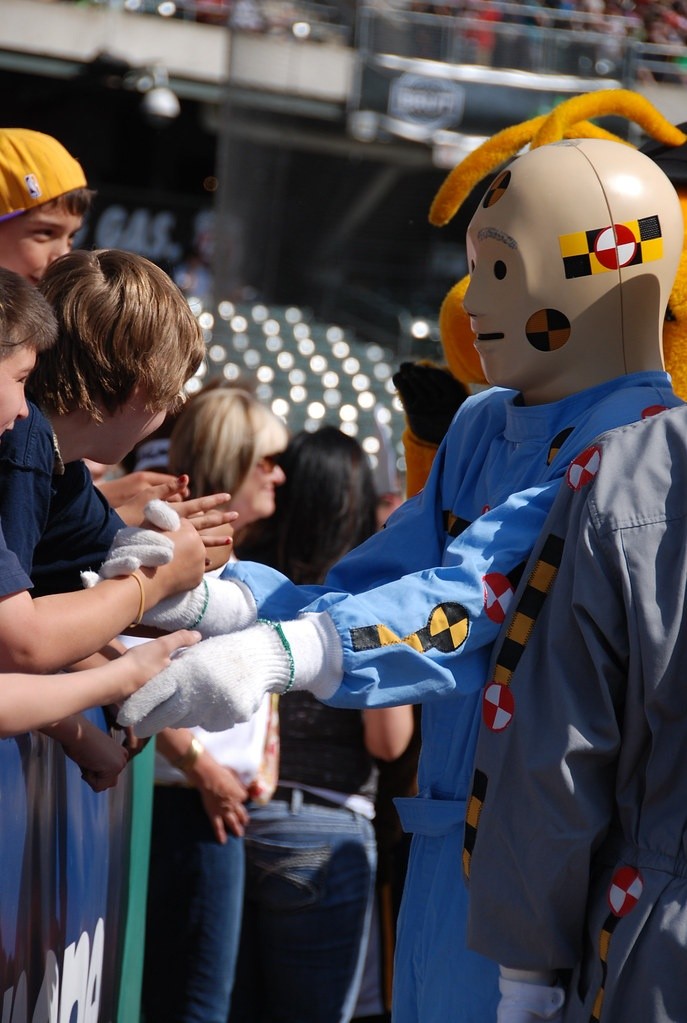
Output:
[387,85,685,500]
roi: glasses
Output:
[259,455,278,473]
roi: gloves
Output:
[116,612,344,738]
[497,977,565,1023]
[81,498,258,634]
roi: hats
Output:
[1,127,87,222]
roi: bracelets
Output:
[122,570,146,630]
[170,738,203,770]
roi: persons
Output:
[138,389,292,1019]
[229,421,417,1022]
[2,247,205,600]
[1,265,211,677]
[81,135,687,1023]
[461,405,686,1023]
[113,476,243,566]
[1,126,191,509]
